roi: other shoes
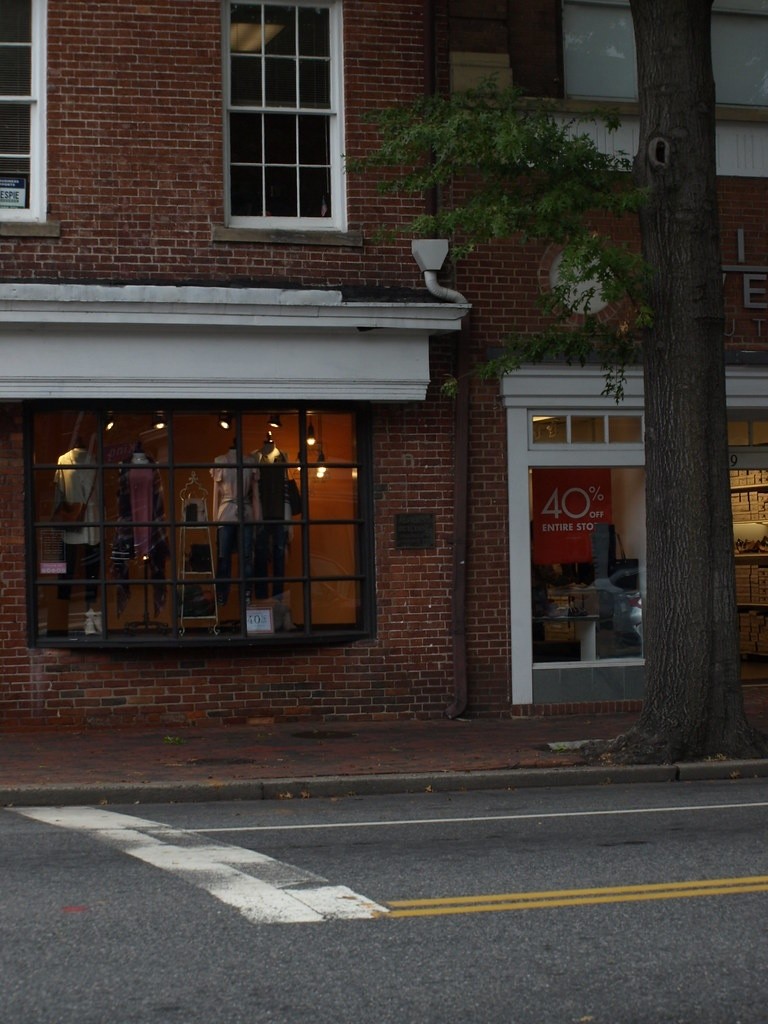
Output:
[84,618,97,635]
[736,536,768,553]
[273,607,282,630]
[84,608,102,633]
[282,608,297,631]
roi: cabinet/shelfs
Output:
[730,484,768,656]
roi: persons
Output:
[49,447,110,602]
[113,451,169,618]
[249,441,293,600]
[212,449,261,607]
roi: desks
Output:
[532,615,600,661]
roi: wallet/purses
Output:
[186,503,197,521]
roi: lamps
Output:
[101,414,115,432]
[317,416,327,471]
[216,416,234,430]
[306,416,316,446]
[152,416,166,430]
[267,415,282,428]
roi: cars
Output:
[612,589,643,646]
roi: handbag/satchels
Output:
[288,477,302,515]
[606,620,642,657]
[177,584,215,617]
[189,544,212,572]
[50,500,86,532]
[608,534,639,589]
